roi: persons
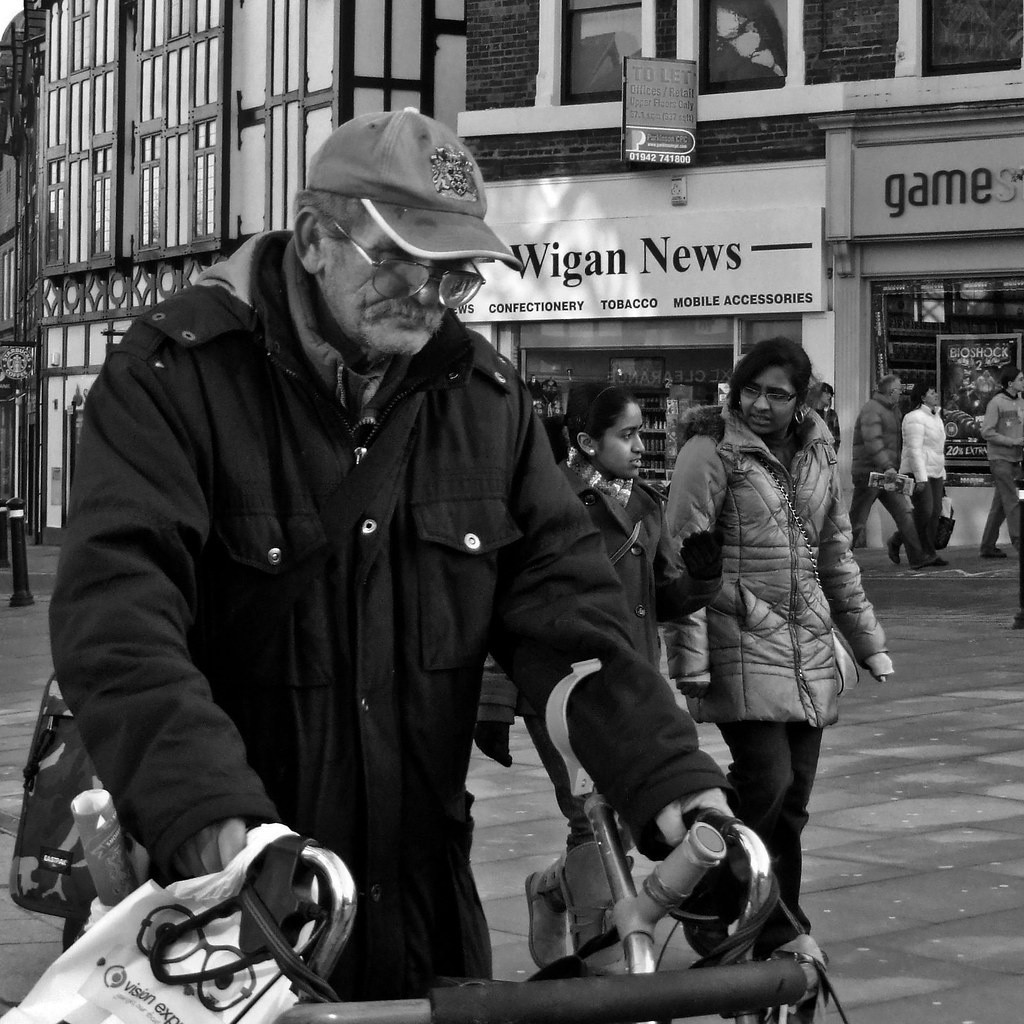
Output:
[50,105,738,1003]
[474,383,727,866]
[808,382,842,455]
[979,370,1024,558]
[848,375,941,572]
[663,336,893,966]
[887,381,949,564]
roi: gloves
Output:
[680,529,724,581]
[473,721,514,768]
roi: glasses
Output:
[317,204,486,308]
[740,385,797,403]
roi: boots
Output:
[886,531,902,563]
[524,854,570,969]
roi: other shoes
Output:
[683,919,729,954]
[980,549,1006,557]
[914,557,947,569]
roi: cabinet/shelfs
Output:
[631,388,670,480]
[889,321,998,365]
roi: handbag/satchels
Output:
[1,821,321,1024]
[935,490,958,549]
[9,671,148,917]
[830,627,859,697]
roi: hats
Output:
[306,106,525,273]
